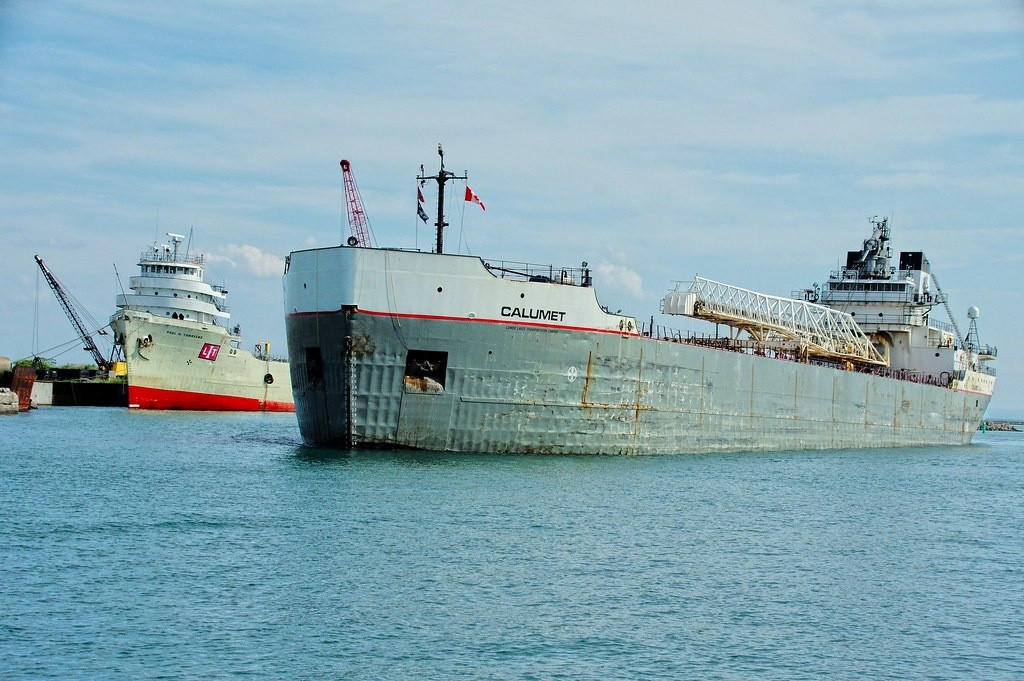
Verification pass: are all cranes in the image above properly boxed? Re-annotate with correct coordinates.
[34,255,116,373]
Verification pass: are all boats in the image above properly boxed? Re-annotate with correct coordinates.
[113,227,295,412]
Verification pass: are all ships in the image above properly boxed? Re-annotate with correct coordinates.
[281,143,999,455]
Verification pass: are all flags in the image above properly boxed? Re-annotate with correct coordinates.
[417,186,429,224]
[464,186,486,211]
